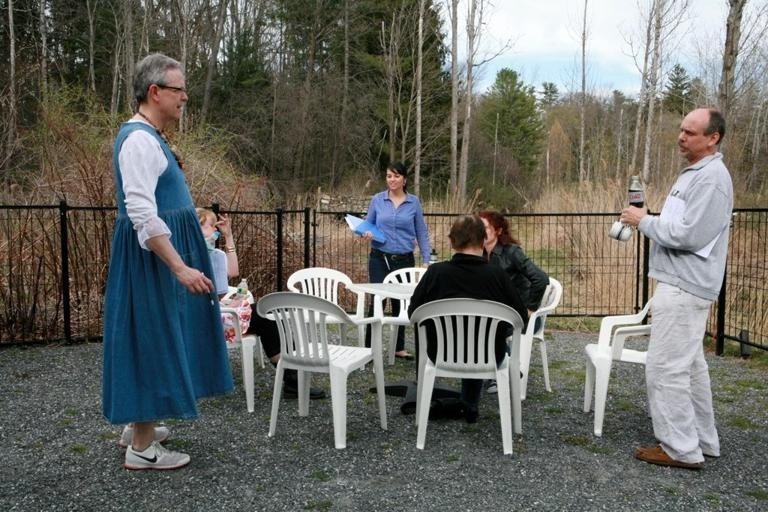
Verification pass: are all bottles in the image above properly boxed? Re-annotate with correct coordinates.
[628,174,645,208]
[237,278,248,298]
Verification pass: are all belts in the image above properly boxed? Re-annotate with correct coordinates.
[370,248,412,262]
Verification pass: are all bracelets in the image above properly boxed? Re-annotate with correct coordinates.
[225,245,235,254]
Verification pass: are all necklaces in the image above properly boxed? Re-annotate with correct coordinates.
[138,111,186,170]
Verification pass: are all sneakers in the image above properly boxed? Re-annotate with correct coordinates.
[633,445,704,470]
[123,442,191,471]
[118,426,171,448]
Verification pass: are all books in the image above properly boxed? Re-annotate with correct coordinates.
[344,213,387,244]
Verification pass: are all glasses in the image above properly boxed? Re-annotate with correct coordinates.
[157,82,189,94]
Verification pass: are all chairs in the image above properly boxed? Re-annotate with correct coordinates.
[519,278,563,402]
[372,268,427,374]
[410,297,524,455]
[287,267,366,372]
[220,288,266,413]
[583,298,650,439]
[256,293,387,451]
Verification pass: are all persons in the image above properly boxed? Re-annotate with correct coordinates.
[407,213,527,423]
[360,161,430,366]
[621,108,735,470]
[193,207,326,400]
[100,55,235,471]
[479,210,550,337]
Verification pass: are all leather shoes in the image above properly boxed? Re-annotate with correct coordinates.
[465,405,479,424]
[397,351,415,360]
[282,384,326,402]
[428,399,467,422]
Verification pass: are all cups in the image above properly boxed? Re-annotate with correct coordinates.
[607,219,633,241]
